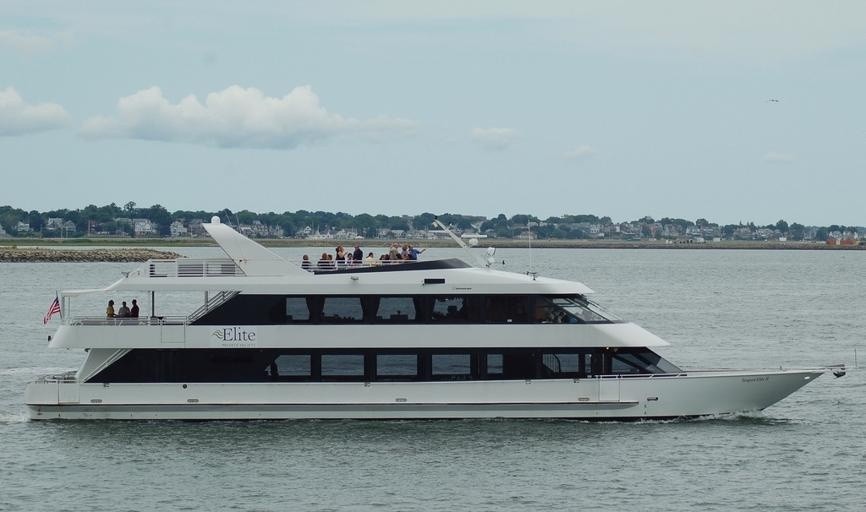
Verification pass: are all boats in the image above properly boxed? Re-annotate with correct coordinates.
[20,214,824,426]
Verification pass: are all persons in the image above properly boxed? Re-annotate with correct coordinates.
[106,300,116,317]
[317,241,426,269]
[118,302,130,317]
[301,255,313,271]
[130,299,139,317]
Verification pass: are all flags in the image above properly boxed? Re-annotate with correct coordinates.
[43,297,61,324]
